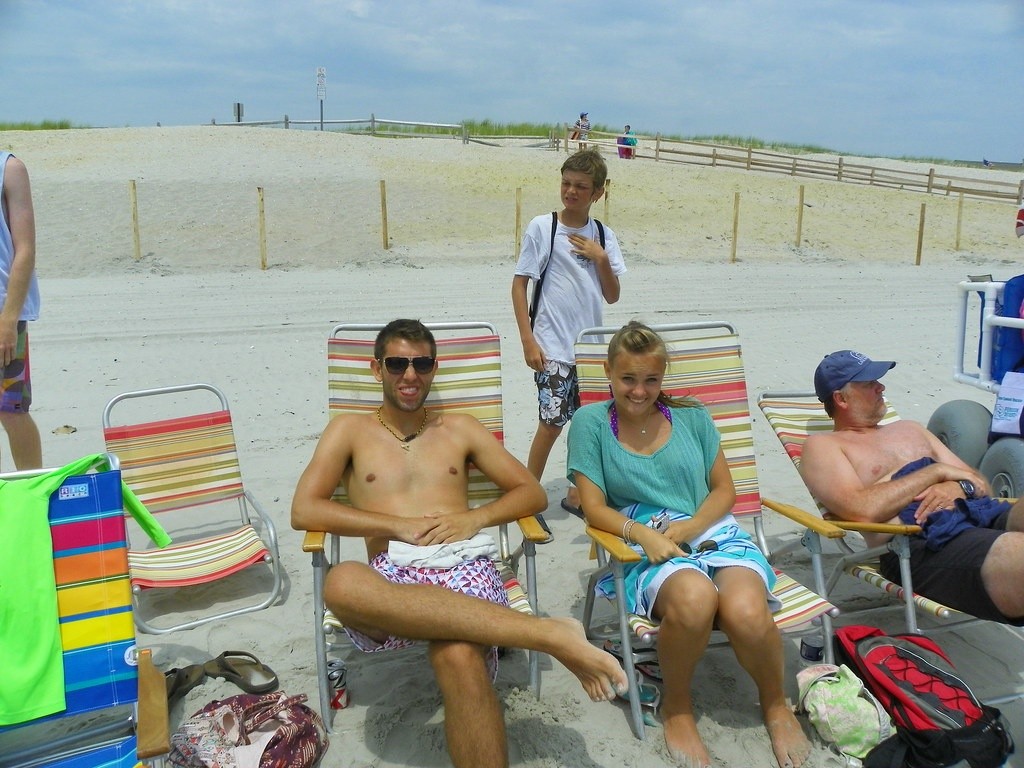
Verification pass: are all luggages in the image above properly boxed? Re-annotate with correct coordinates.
[616,136,632,159]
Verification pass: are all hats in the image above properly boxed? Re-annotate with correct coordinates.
[814,350,896,403]
[580,112,588,117]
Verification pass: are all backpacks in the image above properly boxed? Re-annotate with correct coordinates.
[832,623,1015,768]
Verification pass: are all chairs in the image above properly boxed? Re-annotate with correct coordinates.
[304,322,555,736]
[103,383,280,634]
[0,454,170,768]
[757,390,1024,705]
[575,321,845,741]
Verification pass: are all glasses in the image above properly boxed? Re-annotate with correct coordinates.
[678,540,747,560]
[378,356,437,374]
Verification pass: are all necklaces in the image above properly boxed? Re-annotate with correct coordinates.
[377,404,428,452]
[618,406,651,434]
[627,521,641,546]
[623,519,635,543]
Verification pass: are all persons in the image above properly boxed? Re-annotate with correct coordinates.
[574,112,591,149]
[623,125,636,159]
[801,350,1024,627]
[567,320,812,768]
[512,150,627,545]
[291,318,630,768]
[0,152,43,472]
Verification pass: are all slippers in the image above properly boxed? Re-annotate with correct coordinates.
[164,663,206,711]
[533,514,554,545]
[203,651,279,693]
[606,638,663,680]
[561,497,586,520]
[610,669,662,727]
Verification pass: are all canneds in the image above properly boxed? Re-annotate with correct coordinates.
[326,658,349,709]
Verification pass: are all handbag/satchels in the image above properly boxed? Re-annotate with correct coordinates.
[967,270,1024,385]
[168,687,330,768]
[570,130,580,140]
[986,368,1024,444]
[627,132,638,144]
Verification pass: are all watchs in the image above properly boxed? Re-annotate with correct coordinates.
[957,480,976,500]
